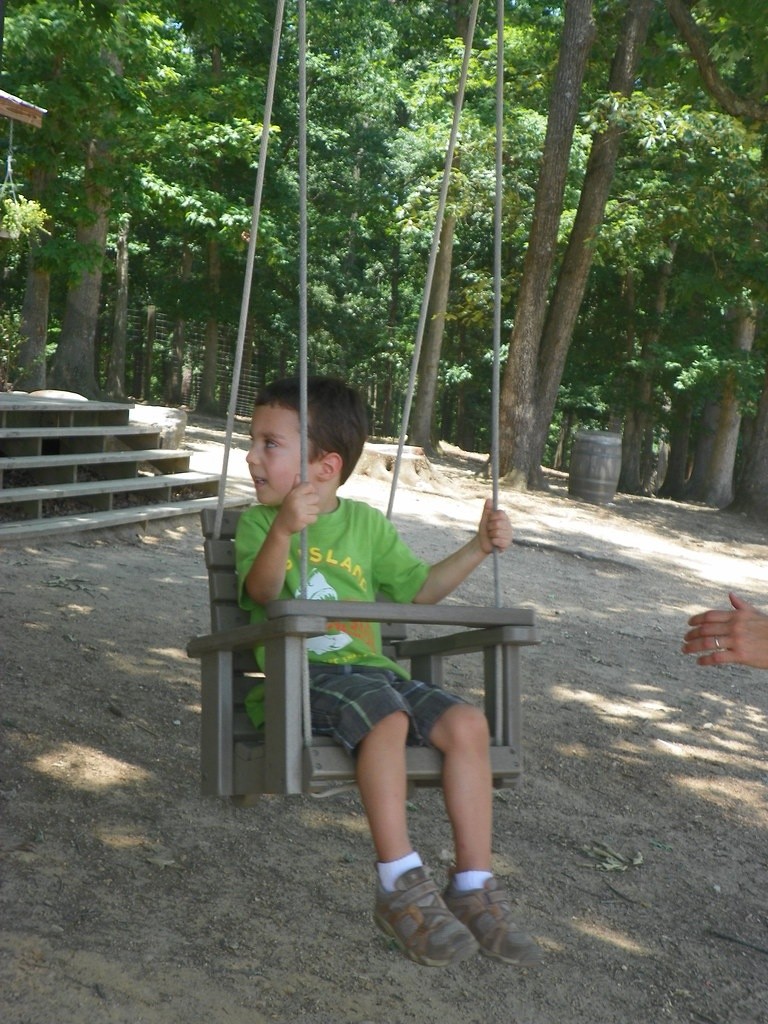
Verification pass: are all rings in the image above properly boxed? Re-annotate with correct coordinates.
[714,636,721,650]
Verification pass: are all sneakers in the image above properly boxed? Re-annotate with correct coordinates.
[372,861,480,968]
[442,866,541,967]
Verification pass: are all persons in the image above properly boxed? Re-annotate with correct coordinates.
[681,591,768,670]
[236,377,543,967]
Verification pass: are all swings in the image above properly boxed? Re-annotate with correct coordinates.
[182,2,541,803]
